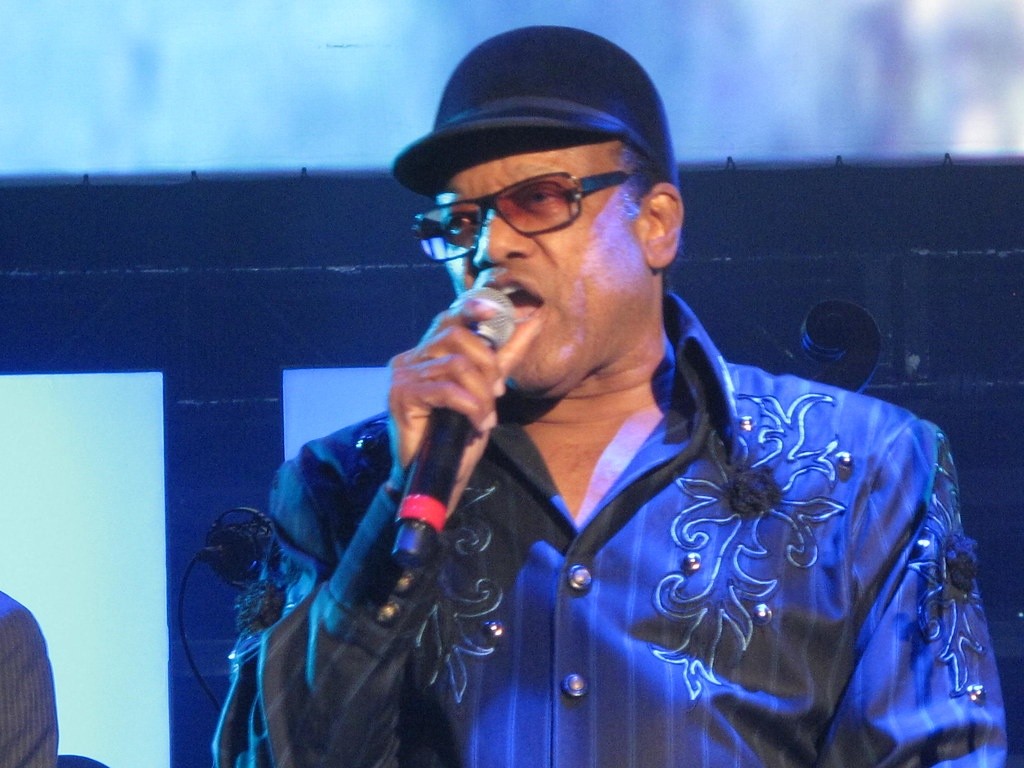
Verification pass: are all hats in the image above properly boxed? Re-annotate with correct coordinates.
[392,24,680,198]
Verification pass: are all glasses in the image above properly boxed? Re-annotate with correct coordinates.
[408,171,667,265]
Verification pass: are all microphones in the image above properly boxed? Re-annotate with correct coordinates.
[391,286,516,569]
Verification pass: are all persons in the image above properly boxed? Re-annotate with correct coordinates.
[212,26,1008,768]
